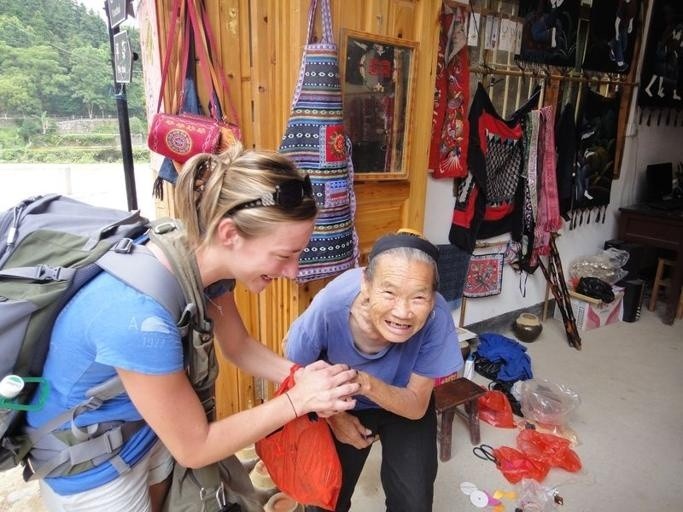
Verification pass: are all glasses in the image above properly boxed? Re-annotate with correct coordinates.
[223,172,313,217]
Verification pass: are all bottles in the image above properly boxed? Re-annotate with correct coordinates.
[234,441,305,512]
[0,374,23,441]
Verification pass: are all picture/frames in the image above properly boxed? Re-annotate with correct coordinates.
[335,27,420,181]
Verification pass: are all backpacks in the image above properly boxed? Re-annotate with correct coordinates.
[0,192,198,482]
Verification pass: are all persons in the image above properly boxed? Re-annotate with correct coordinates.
[277,229,464,512]
[24,147,362,512]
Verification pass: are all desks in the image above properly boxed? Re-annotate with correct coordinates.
[618,203,682,327]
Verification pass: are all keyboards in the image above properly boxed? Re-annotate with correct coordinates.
[648,198,680,210]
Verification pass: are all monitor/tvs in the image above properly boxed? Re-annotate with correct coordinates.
[647,163,672,200]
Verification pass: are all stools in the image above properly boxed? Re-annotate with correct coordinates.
[434,376,488,462]
[648,257,682,321]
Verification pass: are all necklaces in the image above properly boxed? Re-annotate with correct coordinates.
[204,287,225,317]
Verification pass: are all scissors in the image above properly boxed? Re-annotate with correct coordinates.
[472,445,499,466]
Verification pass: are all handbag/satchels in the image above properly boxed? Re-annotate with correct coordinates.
[144,111,242,165]
[165,454,264,512]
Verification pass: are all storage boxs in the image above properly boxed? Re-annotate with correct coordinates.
[553,283,626,331]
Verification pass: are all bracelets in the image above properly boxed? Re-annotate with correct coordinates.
[285,391,298,420]
